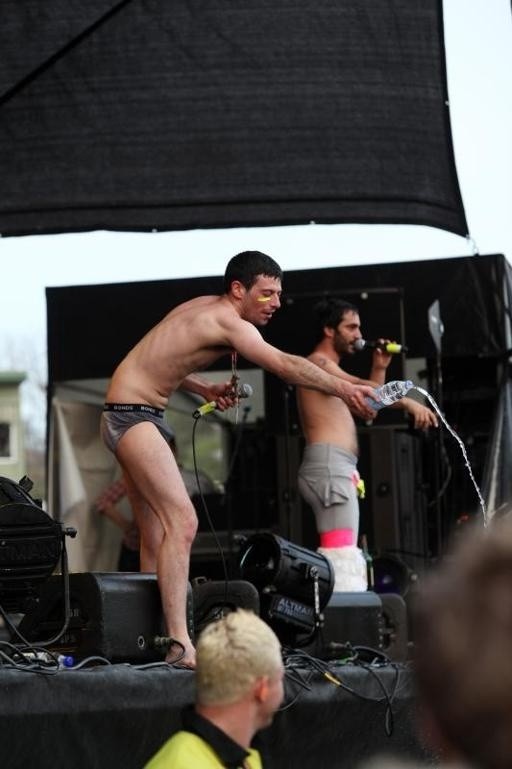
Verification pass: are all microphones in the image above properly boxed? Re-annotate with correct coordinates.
[352,335,412,361]
[190,383,253,419]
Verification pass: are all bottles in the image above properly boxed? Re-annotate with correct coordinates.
[366,380,413,409]
[359,534,374,589]
[13,652,73,668]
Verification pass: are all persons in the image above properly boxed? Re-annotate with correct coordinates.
[294,299,438,549]
[97,470,140,572]
[403,515,512,769]
[141,610,286,769]
[99,251,380,672]
[167,437,217,496]
